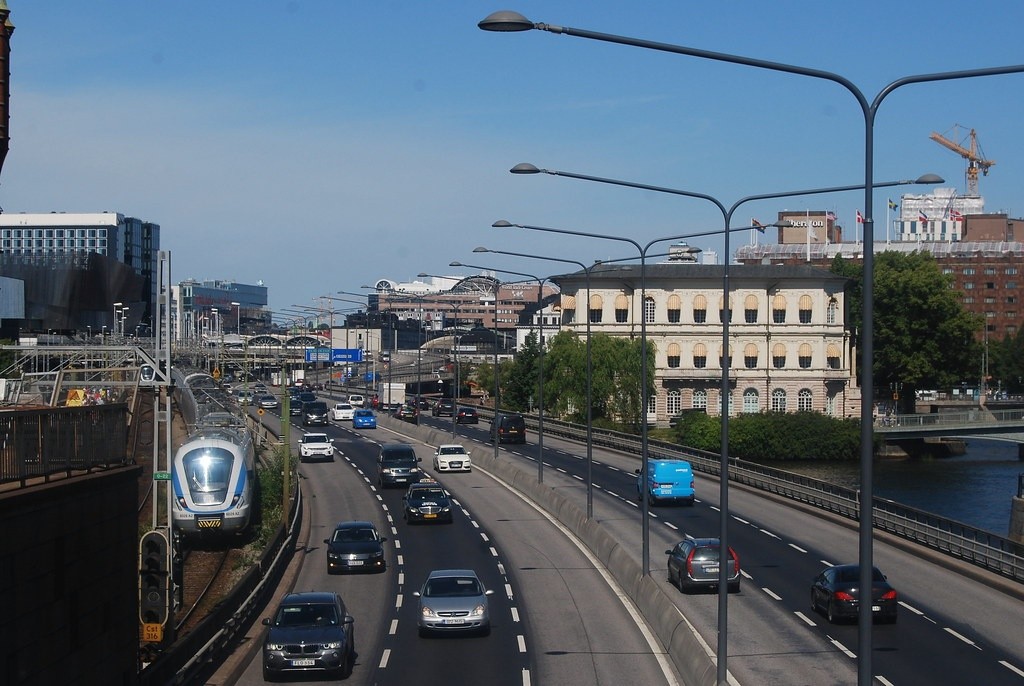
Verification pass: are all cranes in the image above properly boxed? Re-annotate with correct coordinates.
[929,115,994,200]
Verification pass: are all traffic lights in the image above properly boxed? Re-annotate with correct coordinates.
[138,530,170,628]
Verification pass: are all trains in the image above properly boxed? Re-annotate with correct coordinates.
[163,360,258,546]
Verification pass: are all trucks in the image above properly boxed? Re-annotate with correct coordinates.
[376,382,407,413]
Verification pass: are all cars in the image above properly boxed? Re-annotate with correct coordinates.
[413,569,495,639]
[258,394,278,410]
[261,590,356,682]
[237,390,254,406]
[297,432,334,463]
[664,536,741,595]
[433,443,472,473]
[810,561,899,626]
[329,404,357,421]
[392,404,420,424]
[348,395,364,408]
[401,477,455,524]
[351,409,378,429]
[322,520,386,576]
[407,397,429,411]
[453,407,478,424]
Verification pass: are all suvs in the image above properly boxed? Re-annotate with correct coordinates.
[431,398,455,417]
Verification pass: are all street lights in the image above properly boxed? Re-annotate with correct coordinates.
[448,261,633,486]
[478,7,1024,686]
[507,160,946,686]
[202,284,525,440]
[417,272,569,460]
[493,215,793,577]
[472,245,702,522]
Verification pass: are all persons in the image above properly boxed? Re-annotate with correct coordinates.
[479,397,484,405]
[486,394,489,400]
[988,387,999,401]
[873,402,879,422]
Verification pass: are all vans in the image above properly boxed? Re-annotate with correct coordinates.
[488,413,526,444]
[634,458,695,507]
[376,443,423,489]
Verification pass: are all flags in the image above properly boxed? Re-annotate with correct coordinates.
[889,198,898,212]
[827,211,839,221]
[950,208,964,221]
[856,211,865,223]
[810,224,818,241]
[919,210,927,222]
[752,218,766,234]
